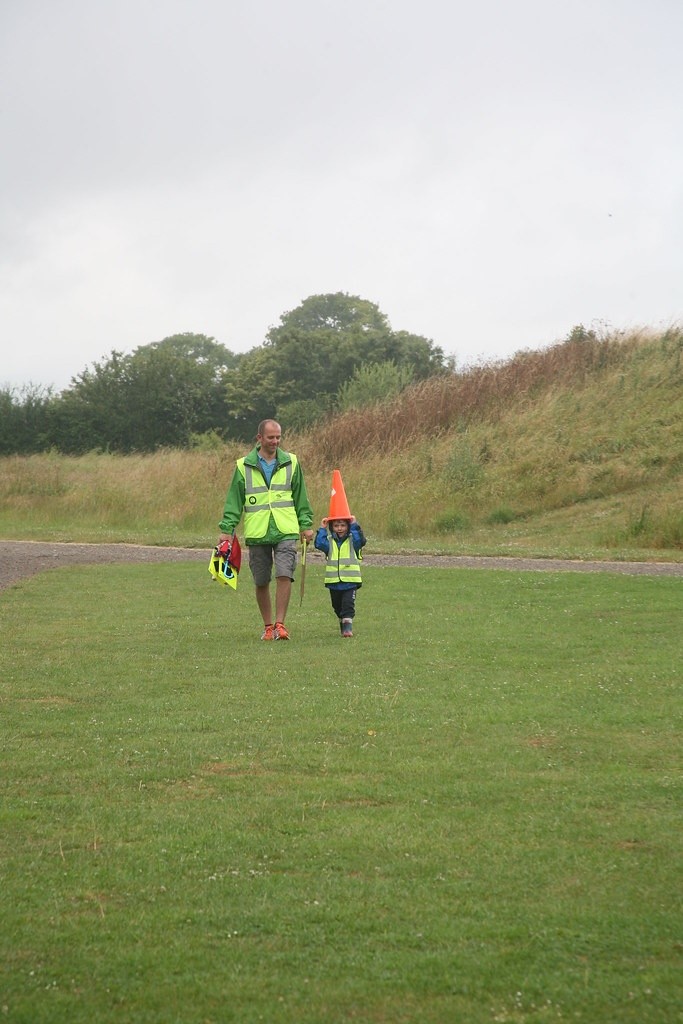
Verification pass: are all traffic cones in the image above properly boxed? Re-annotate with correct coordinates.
[323,469,352,521]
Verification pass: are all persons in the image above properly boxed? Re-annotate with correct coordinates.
[314,515,368,639]
[216,418,314,642]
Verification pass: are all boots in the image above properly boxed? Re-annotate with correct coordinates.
[338,617,353,637]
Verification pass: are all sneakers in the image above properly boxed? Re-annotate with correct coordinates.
[261,624,274,641]
[274,622,290,640]
